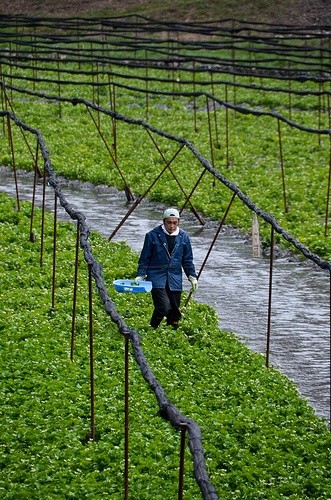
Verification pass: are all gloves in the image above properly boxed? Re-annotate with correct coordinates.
[189,276,198,292]
[135,276,146,284]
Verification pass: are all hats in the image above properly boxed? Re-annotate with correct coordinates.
[164,208,179,219]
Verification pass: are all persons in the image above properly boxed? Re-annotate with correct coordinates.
[135,208,199,330]
